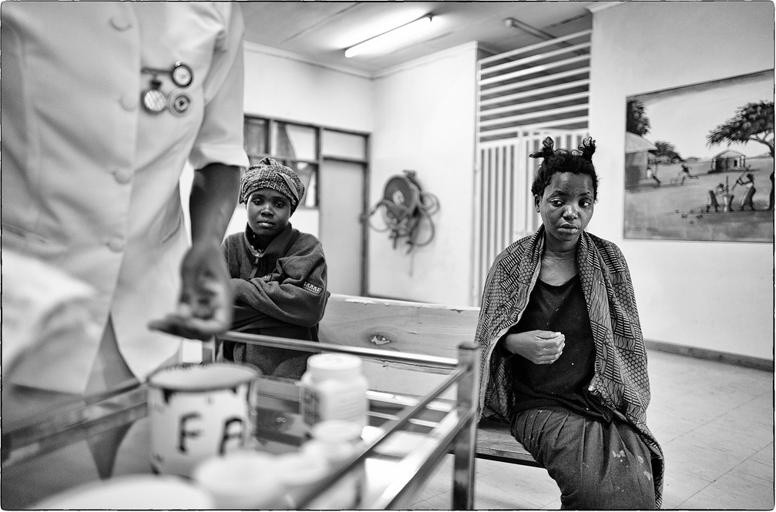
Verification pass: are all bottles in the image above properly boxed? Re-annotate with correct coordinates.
[296,351,369,443]
[191,417,368,509]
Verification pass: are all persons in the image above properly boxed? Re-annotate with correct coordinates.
[215,155,329,388]
[0,0,248,509]
[474,142,667,510]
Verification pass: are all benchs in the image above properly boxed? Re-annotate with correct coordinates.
[237,294,549,468]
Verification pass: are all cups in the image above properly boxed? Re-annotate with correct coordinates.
[144,359,262,480]
